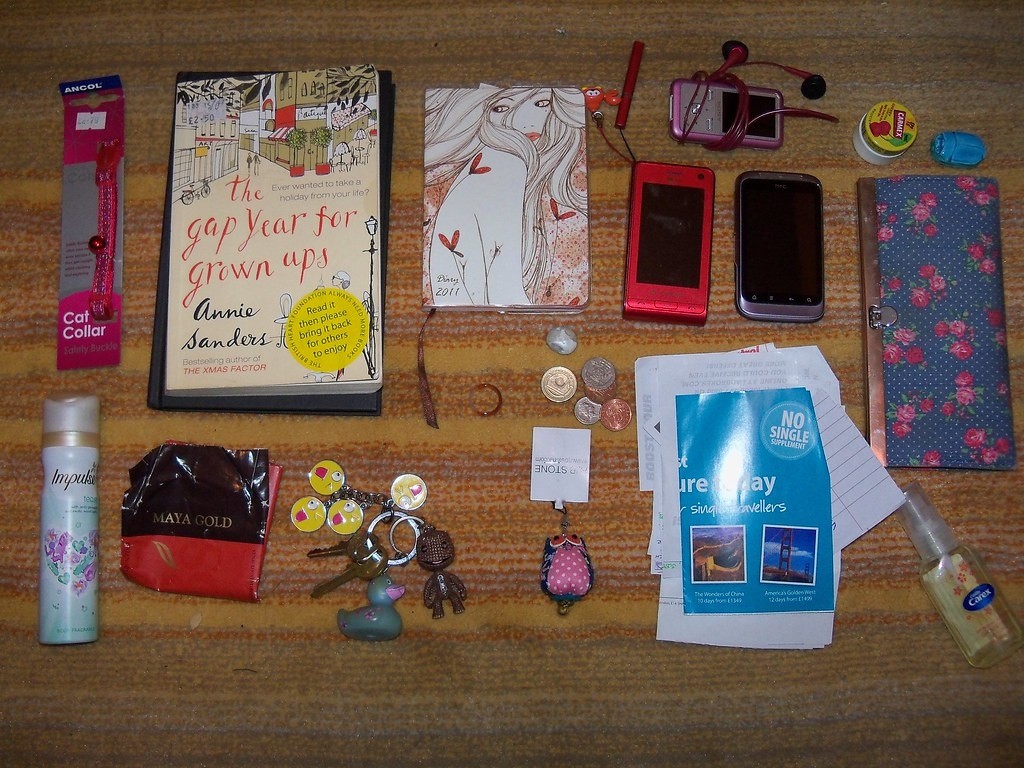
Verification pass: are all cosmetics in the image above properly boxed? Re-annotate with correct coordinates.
[895,482,1024,669]
[37,394,101,643]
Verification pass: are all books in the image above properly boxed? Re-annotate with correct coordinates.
[422,85,588,311]
[148,64,395,416]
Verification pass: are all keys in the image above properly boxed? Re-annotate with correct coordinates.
[307,532,379,563]
[311,546,388,599]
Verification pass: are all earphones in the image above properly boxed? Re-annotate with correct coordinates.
[720,40,749,75]
[784,65,826,99]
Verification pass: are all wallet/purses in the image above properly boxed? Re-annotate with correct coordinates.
[856,172,1017,472]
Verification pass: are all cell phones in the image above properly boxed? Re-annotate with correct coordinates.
[734,169,824,323]
[622,160,716,328]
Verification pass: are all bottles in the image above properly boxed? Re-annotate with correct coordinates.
[894,480,1024,667]
